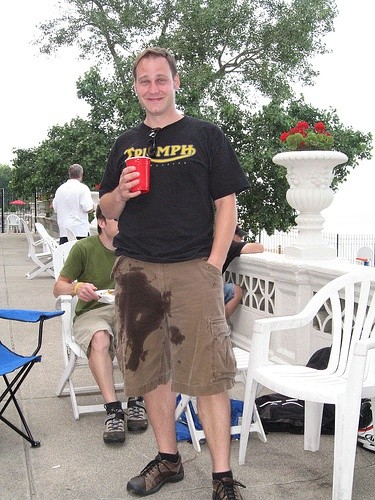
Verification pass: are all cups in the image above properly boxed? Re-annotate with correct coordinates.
[125,156,151,194]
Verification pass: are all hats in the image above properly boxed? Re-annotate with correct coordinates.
[235,225,247,236]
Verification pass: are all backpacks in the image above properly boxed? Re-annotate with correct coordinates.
[258,347,373,435]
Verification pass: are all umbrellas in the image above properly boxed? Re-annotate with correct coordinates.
[10,200,27,212]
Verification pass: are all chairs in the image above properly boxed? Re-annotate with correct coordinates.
[240,265,375,500]
[54,240,125,421]
[20,218,60,279]
[7,214,21,234]
[0,310,65,449]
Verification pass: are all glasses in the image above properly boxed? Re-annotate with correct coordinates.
[147,126,161,156]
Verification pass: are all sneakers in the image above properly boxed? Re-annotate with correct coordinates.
[127,394,147,430]
[103,406,126,443]
[126,454,185,496]
[211,478,246,500]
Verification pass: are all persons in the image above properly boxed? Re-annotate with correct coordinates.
[52,205,147,444]
[51,164,95,244]
[99,47,249,500]
[222,226,265,328]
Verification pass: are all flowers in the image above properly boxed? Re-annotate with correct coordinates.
[279,122,334,149]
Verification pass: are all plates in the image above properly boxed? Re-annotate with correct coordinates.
[94,289,115,299]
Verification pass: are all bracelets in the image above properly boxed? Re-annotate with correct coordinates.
[73,282,83,295]
